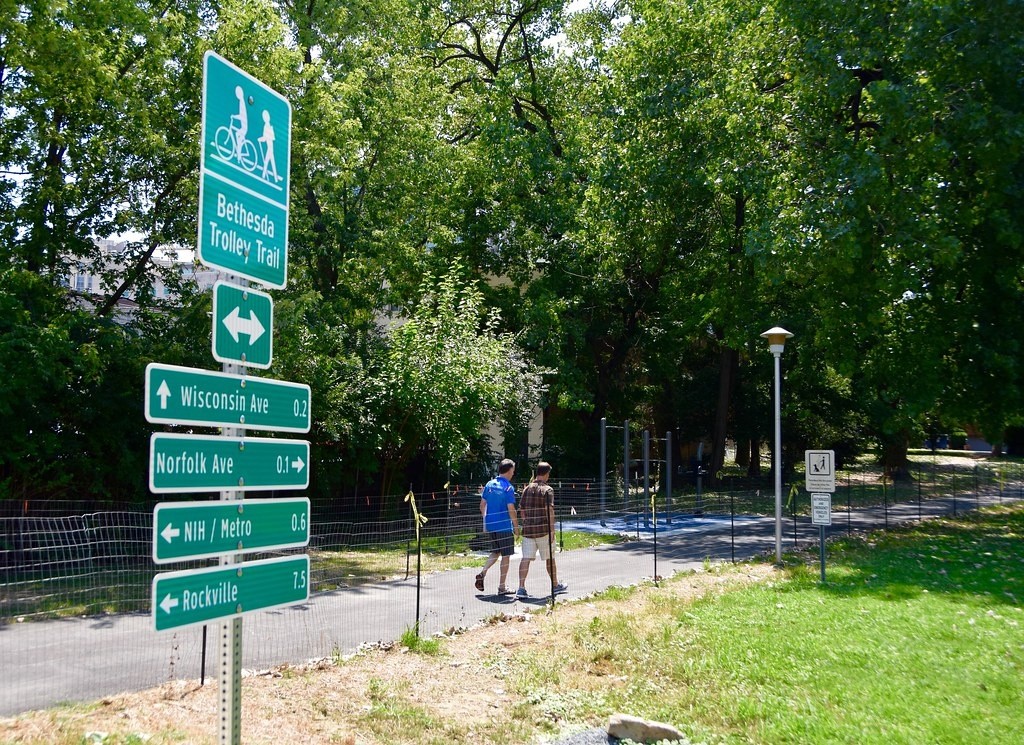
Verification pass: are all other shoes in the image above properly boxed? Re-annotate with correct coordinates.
[498,585,516,595]
[475,573,485,591]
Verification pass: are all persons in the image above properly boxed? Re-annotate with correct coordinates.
[475,459,520,595]
[514,463,568,599]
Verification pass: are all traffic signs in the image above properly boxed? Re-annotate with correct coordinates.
[144,365,312,635]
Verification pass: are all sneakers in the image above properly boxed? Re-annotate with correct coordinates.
[553,583,568,594]
[516,588,533,598]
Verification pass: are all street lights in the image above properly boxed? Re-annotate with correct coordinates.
[759,326,795,563]
